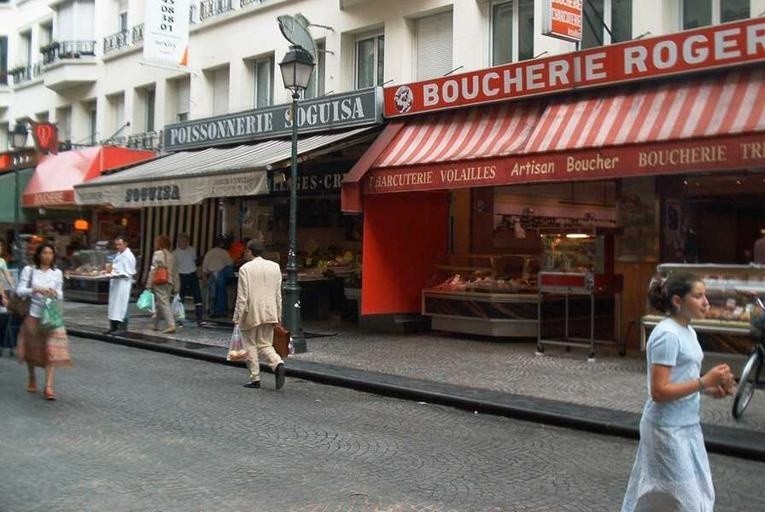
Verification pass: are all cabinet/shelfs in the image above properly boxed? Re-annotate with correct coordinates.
[641,261,765,384]
[62,247,144,305]
[420,253,540,342]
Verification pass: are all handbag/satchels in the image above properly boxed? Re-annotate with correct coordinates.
[40,295,63,329]
[7,295,32,317]
[154,267,168,285]
[273,326,290,357]
[137,289,152,310]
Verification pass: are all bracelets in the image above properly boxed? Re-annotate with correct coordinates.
[696,376,705,393]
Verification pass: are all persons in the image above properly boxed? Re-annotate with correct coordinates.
[14,243,72,400]
[623,272,739,511]
[0,230,248,357]
[231,237,286,389]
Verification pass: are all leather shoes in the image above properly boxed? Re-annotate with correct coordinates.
[243,383,260,388]
[276,363,286,389]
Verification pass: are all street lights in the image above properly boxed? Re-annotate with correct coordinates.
[277,45,317,355]
[5,123,29,269]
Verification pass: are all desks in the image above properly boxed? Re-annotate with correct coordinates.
[297,276,338,320]
[537,271,624,360]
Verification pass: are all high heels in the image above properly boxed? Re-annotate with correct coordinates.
[28,383,37,392]
[44,385,56,399]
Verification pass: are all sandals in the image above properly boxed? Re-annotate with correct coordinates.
[164,327,176,333]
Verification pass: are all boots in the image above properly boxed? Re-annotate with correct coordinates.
[110,319,126,335]
[195,305,207,327]
[103,319,120,334]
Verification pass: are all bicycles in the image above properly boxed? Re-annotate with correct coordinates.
[730,284,764,422]
